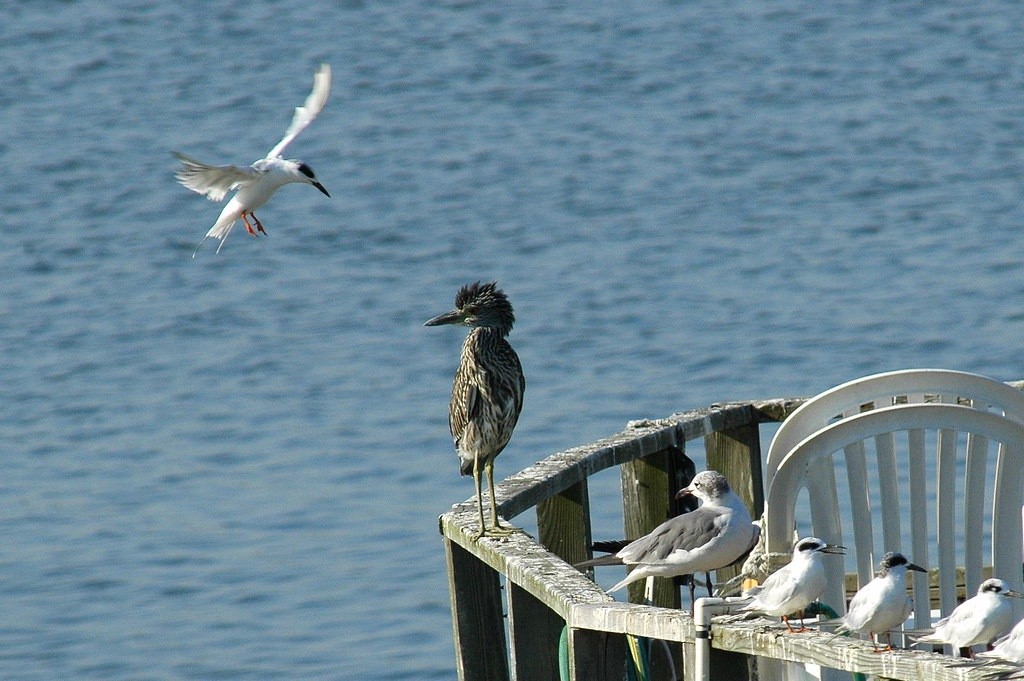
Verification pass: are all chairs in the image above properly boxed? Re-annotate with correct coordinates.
[761,369,1024,680]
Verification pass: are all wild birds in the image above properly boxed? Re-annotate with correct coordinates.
[906,578,1024,681]
[172,63,331,259]
[824,551,928,652]
[423,280,535,543]
[573,470,849,634]
